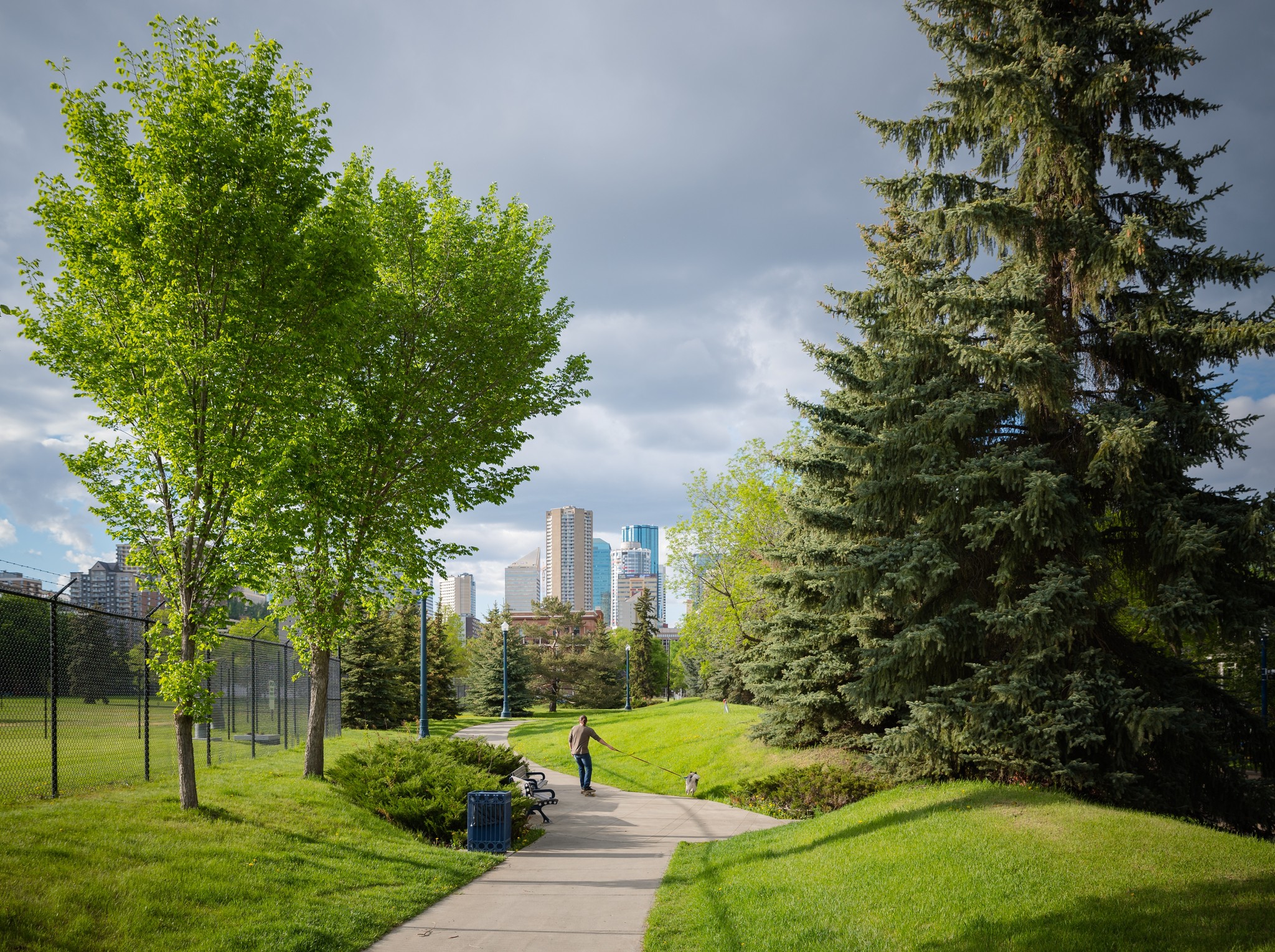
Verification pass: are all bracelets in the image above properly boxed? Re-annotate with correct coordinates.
[571,750,572,751]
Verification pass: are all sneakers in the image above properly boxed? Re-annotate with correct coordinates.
[581,787,595,792]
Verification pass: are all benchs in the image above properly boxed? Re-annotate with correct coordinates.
[508,764,559,824]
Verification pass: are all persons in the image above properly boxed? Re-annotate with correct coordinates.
[568,715,613,792]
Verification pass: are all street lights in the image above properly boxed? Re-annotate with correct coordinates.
[624,644,632,710]
[501,621,512,717]
[415,574,432,741]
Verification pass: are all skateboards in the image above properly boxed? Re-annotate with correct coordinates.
[579,786,596,796]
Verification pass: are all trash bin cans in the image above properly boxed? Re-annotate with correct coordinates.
[468,790,511,853]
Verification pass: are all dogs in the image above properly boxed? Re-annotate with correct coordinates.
[684,770,700,796]
